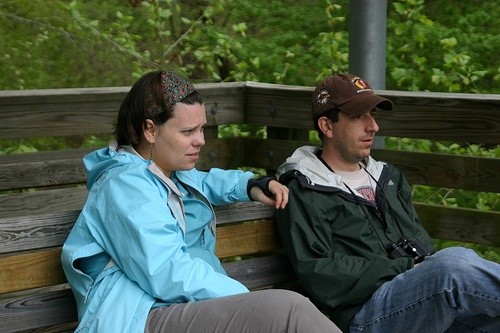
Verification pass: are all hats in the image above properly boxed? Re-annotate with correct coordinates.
[311,74,394,121]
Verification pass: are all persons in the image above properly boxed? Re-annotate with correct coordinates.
[272,73,500,333]
[61,71,343,333]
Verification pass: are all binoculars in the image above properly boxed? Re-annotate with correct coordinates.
[385,235,427,264]
